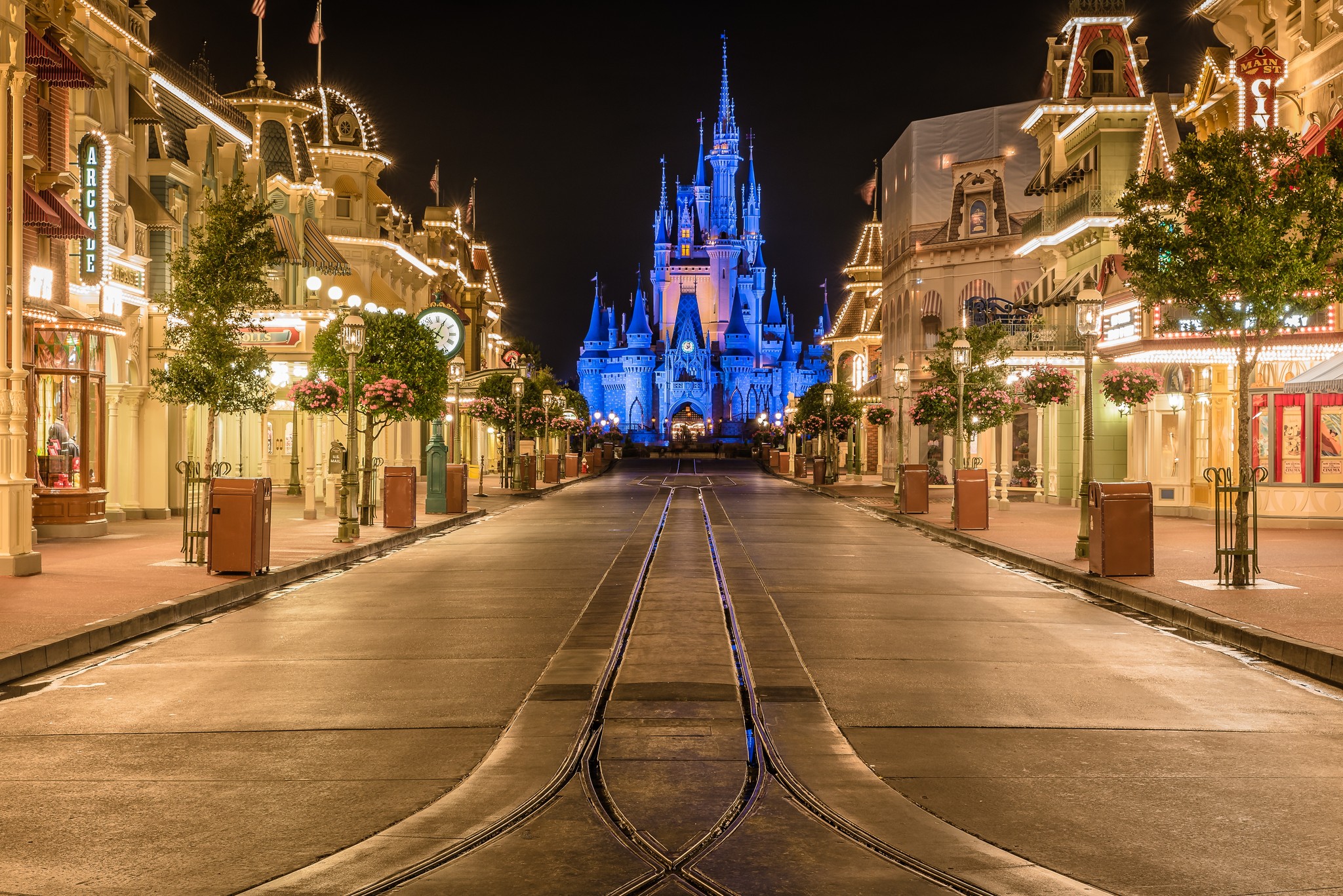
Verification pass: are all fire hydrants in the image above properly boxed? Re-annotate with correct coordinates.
[581,455,590,475]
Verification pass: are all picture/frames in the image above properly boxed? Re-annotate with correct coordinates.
[47,438,61,456]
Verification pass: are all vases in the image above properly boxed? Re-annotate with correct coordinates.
[929,476,934,484]
[1031,484,1036,487]
[1010,484,1021,487]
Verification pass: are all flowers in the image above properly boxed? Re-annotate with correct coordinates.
[928,464,949,485]
[287,354,625,440]
[1010,464,1043,485]
[752,362,1165,442]
[1027,312,1046,326]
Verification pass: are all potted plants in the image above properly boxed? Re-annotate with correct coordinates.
[1018,429,1031,487]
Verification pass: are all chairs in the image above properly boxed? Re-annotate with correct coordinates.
[997,329,1057,351]
[1170,432,1179,476]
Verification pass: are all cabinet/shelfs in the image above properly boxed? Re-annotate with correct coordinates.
[37,456,73,487]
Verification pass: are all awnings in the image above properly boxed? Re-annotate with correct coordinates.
[7,173,95,240]
[921,279,997,319]
[1260,109,1343,197]
[129,83,166,126]
[268,215,352,276]
[128,174,181,231]
[1023,145,1098,196]
[334,269,406,308]
[1003,379,1038,405]
[34,303,125,336]
[1283,351,1343,393]
[24,21,108,90]
[498,358,508,368]
[1012,265,1097,308]
[430,288,471,326]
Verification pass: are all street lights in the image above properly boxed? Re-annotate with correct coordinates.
[1073,272,1107,561]
[445,352,465,465]
[824,384,834,484]
[541,381,555,481]
[506,366,529,489]
[892,355,915,509]
[588,405,620,447]
[665,417,669,431]
[338,304,366,538]
[707,417,711,431]
[757,404,786,448]
[651,416,656,430]
[557,390,567,479]
[948,329,973,524]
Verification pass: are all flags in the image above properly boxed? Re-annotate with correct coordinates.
[251,0,265,18]
[308,9,324,44]
[466,187,473,224]
[430,165,437,193]
[860,170,876,205]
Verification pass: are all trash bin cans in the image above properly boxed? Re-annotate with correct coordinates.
[545,454,561,483]
[382,466,416,528]
[592,448,602,466]
[761,443,770,461]
[614,445,623,459]
[584,452,594,474]
[813,455,827,484]
[794,454,807,478]
[1087,482,1155,578]
[603,442,613,460]
[751,448,760,459]
[519,455,536,489]
[565,453,578,477]
[954,468,989,531]
[899,463,929,515]
[208,476,272,577]
[446,463,468,515]
[770,448,779,466]
[779,452,790,473]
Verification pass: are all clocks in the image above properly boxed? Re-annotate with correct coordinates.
[415,307,466,361]
[681,340,695,353]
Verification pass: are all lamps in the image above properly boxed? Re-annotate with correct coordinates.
[1118,401,1131,418]
[444,414,453,426]
[306,276,407,317]
[651,416,723,428]
[1165,379,1180,414]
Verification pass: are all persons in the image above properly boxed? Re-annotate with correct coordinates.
[48,415,79,458]
[680,422,688,444]
[496,434,504,469]
[714,439,723,459]
